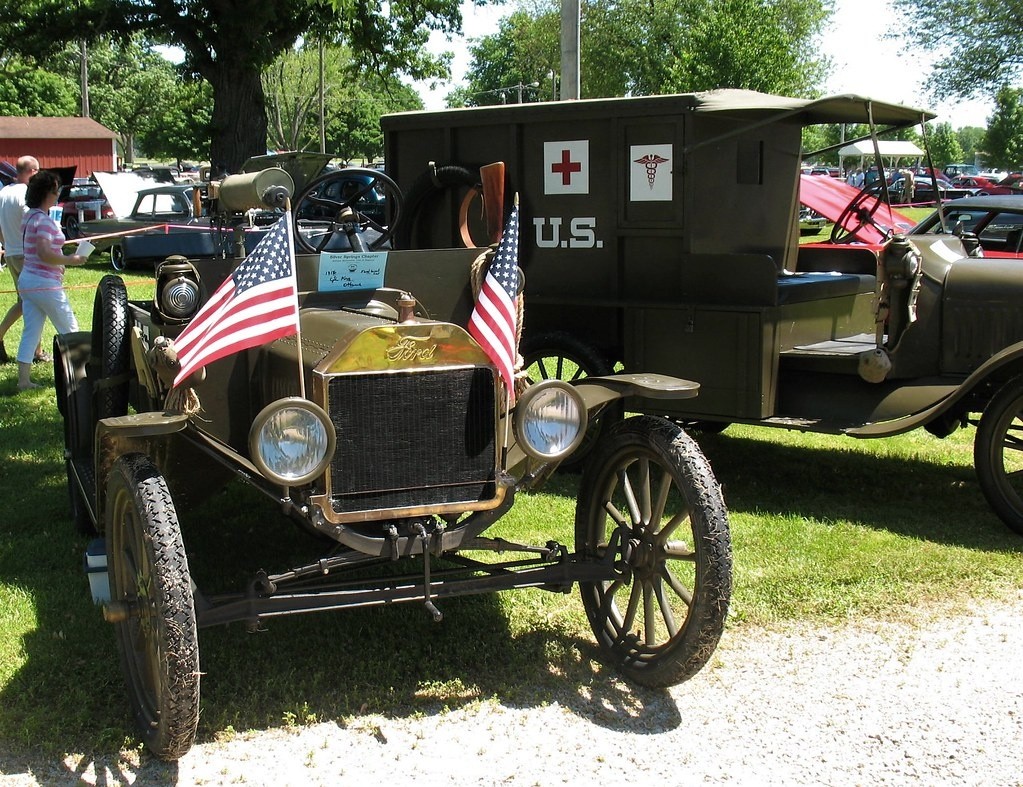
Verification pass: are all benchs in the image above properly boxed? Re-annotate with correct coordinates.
[682,246,879,306]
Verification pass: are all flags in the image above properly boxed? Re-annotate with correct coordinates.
[467,205,520,403]
[173,210,299,388]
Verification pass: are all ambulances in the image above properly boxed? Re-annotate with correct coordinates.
[378,82,1022,534]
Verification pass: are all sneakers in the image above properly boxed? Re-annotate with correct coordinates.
[0,341,9,362]
[33,350,53,363]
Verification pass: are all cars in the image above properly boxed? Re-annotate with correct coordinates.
[122,146,385,191]
[75,183,213,253]
[48,170,736,758]
[901,192,1022,262]
[795,159,1023,201]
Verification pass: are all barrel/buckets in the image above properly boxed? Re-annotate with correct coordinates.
[82,550,112,607]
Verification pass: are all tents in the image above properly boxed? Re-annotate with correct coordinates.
[836,139,924,169]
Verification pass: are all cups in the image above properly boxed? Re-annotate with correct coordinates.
[75,241,96,265]
[50,206,63,227]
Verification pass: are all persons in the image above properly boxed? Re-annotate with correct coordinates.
[16,171,80,389]
[0,155,53,365]
[892,167,914,209]
[843,167,876,187]
